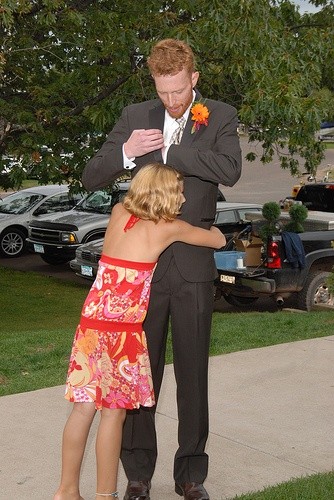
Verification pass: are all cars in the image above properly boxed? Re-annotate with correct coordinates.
[25,179,226,264]
[0,183,84,258]
[69,201,263,283]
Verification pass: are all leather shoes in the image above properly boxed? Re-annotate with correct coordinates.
[123,479,151,500]
[175,481,210,500]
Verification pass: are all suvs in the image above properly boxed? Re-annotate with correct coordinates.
[213,181,334,312]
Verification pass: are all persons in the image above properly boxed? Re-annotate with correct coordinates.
[54,163,226,500]
[81,41,242,500]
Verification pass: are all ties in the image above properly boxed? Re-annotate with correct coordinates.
[169,116,187,145]
